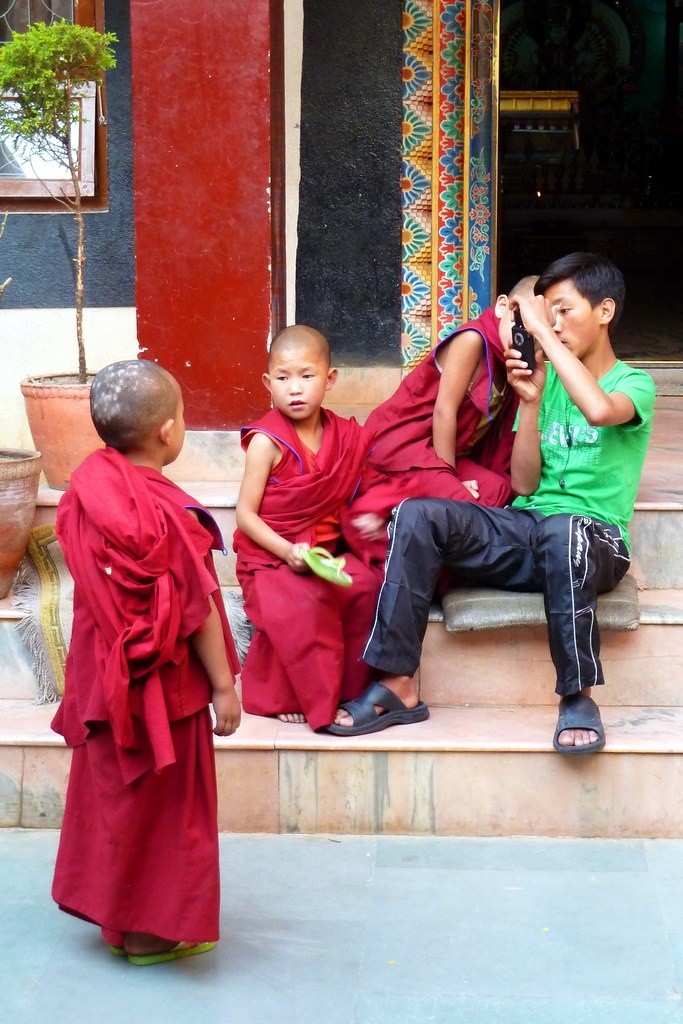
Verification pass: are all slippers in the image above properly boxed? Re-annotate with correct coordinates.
[110,940,219,964]
[554,694,607,756]
[324,680,430,734]
[300,546,353,588]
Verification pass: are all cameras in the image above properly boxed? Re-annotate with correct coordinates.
[509,308,536,370]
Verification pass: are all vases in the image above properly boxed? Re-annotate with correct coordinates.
[0,448,42,600]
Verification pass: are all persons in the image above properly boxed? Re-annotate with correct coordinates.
[309,251,656,759]
[230,326,401,731]
[50,361,242,968]
[362,273,548,506]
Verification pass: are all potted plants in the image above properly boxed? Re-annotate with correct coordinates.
[0,18,119,492]
